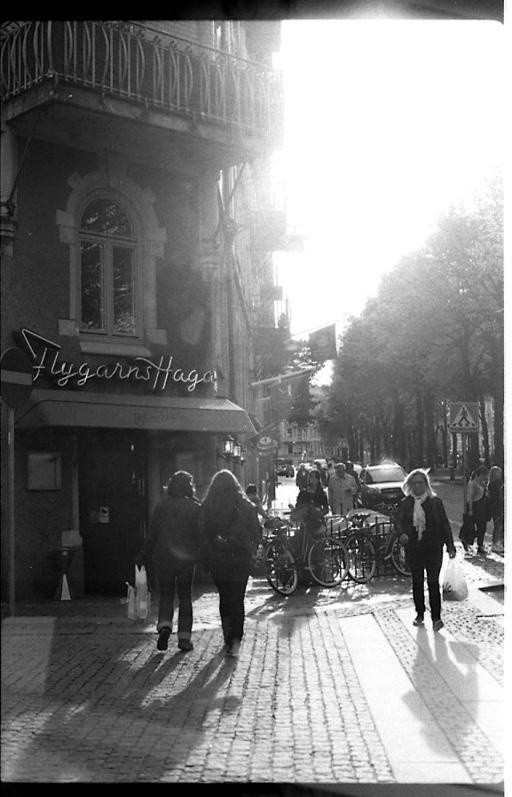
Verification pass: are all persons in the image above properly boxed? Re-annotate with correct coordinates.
[196,468,261,657]
[392,466,457,631]
[136,470,200,651]
[245,480,267,523]
[457,465,491,557]
[486,465,503,552]
[284,455,361,519]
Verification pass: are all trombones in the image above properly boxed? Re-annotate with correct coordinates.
[447,400,481,434]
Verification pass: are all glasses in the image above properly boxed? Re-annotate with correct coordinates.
[217,434,246,467]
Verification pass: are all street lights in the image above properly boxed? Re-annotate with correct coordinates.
[478,504,491,522]
[459,517,475,545]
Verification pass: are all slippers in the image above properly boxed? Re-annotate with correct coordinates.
[202,501,251,569]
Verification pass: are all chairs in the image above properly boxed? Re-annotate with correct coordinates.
[222,639,242,659]
[156,627,171,651]
[177,638,194,652]
[492,543,504,552]
[432,619,444,633]
[465,543,477,555]
[477,545,490,555]
[413,613,424,626]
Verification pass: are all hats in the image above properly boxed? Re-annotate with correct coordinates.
[275,454,413,516]
